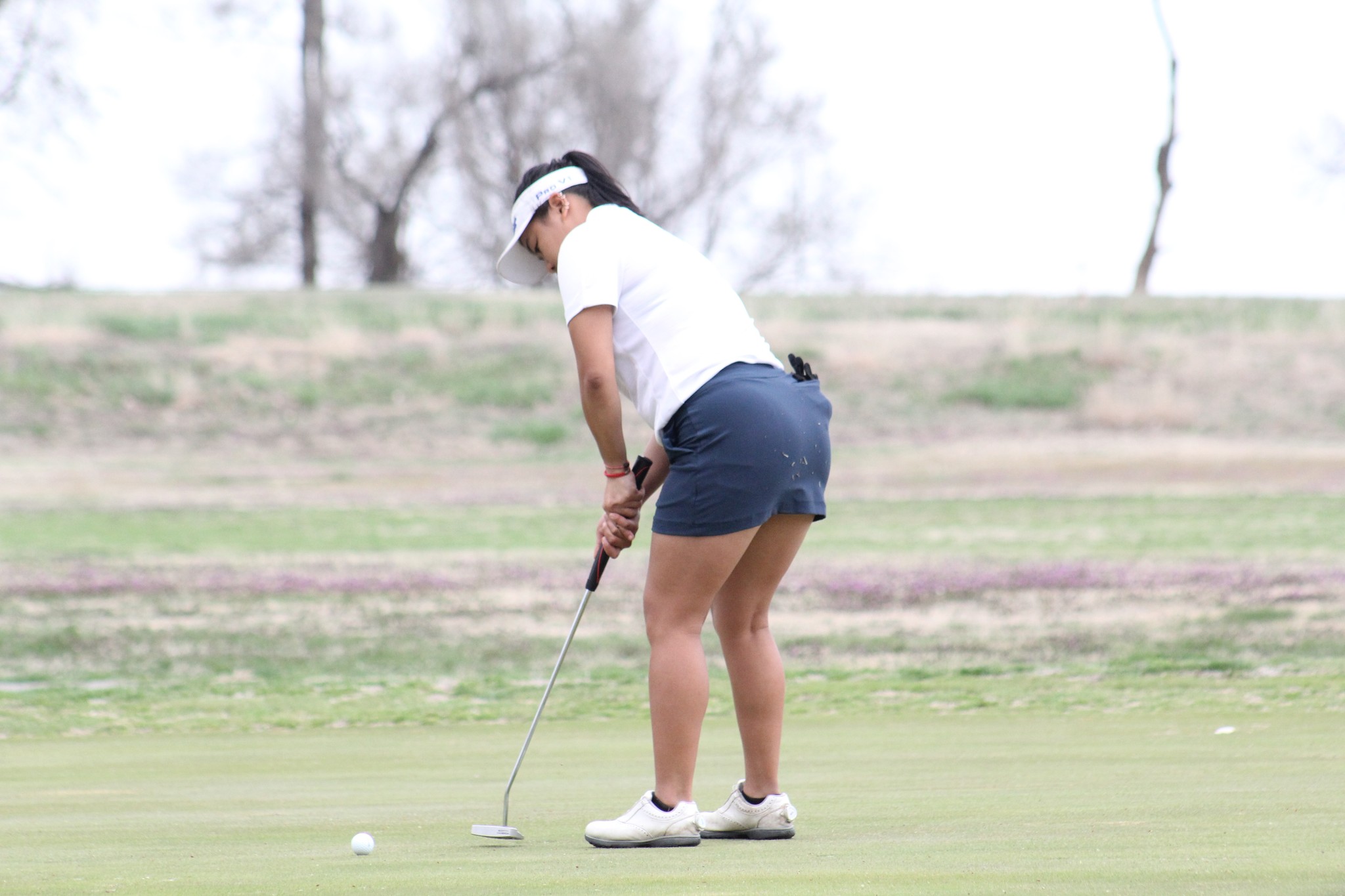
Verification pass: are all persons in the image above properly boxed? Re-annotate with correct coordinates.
[494,151,833,847]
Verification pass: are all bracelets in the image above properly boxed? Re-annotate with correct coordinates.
[604,462,631,478]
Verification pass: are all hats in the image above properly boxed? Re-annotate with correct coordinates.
[496,165,588,286]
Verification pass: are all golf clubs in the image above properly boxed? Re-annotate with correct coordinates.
[469,448,655,844]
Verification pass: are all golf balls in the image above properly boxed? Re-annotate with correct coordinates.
[350,832,375,856]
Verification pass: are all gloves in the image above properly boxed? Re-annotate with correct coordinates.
[788,354,818,382]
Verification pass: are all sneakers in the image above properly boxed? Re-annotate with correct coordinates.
[700,779,797,839]
[584,790,701,848]
